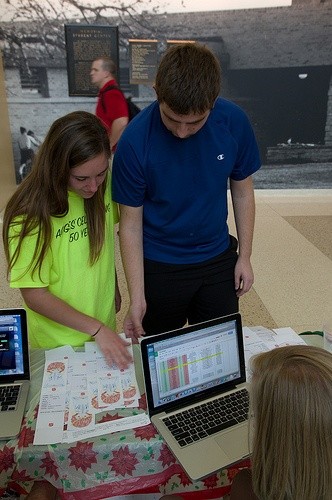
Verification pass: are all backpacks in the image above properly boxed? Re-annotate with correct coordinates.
[101,85,142,126]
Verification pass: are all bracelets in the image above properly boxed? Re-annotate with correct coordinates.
[91,323,104,338]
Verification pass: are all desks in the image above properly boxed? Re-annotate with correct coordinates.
[0,335,332,500]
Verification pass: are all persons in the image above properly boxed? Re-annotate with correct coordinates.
[111,43,262,343]
[2,111,134,371]
[231,345,332,500]
[17,126,42,171]
[90,55,130,161]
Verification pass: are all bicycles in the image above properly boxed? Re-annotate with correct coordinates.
[18,157,32,181]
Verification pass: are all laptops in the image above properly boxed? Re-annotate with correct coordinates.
[140,313,255,483]
[0,309,30,441]
